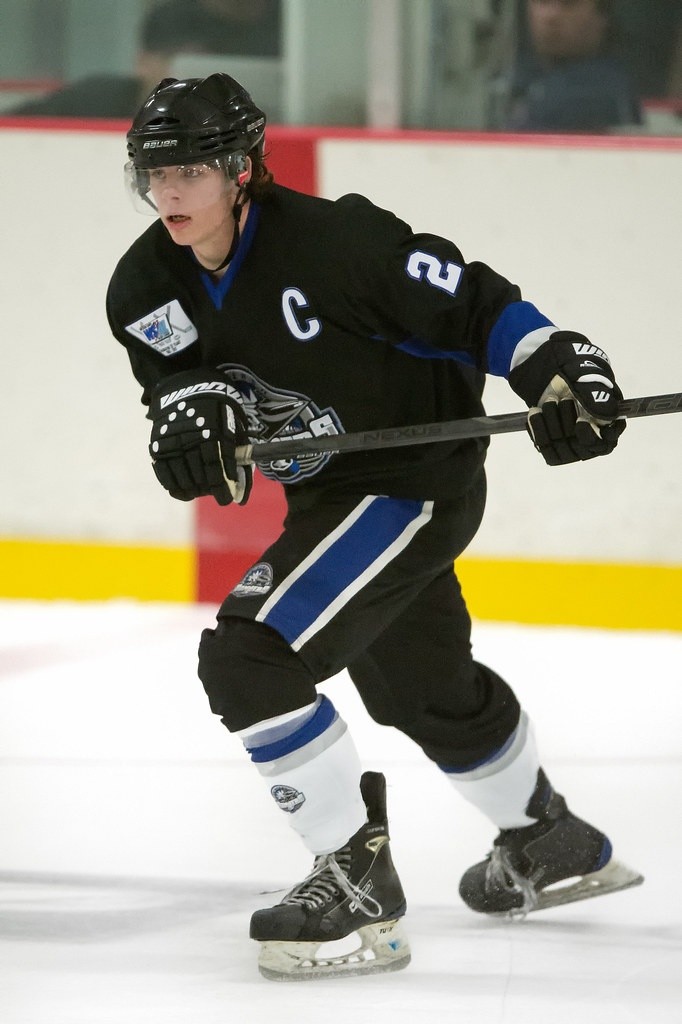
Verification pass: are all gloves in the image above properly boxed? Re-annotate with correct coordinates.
[143,365,257,507]
[506,327,629,466]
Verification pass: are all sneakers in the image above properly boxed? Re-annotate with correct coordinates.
[248,771,413,982]
[458,771,649,920]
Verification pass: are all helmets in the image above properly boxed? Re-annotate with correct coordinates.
[123,68,270,217]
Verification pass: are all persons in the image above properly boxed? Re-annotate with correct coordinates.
[105,73,645,979]
